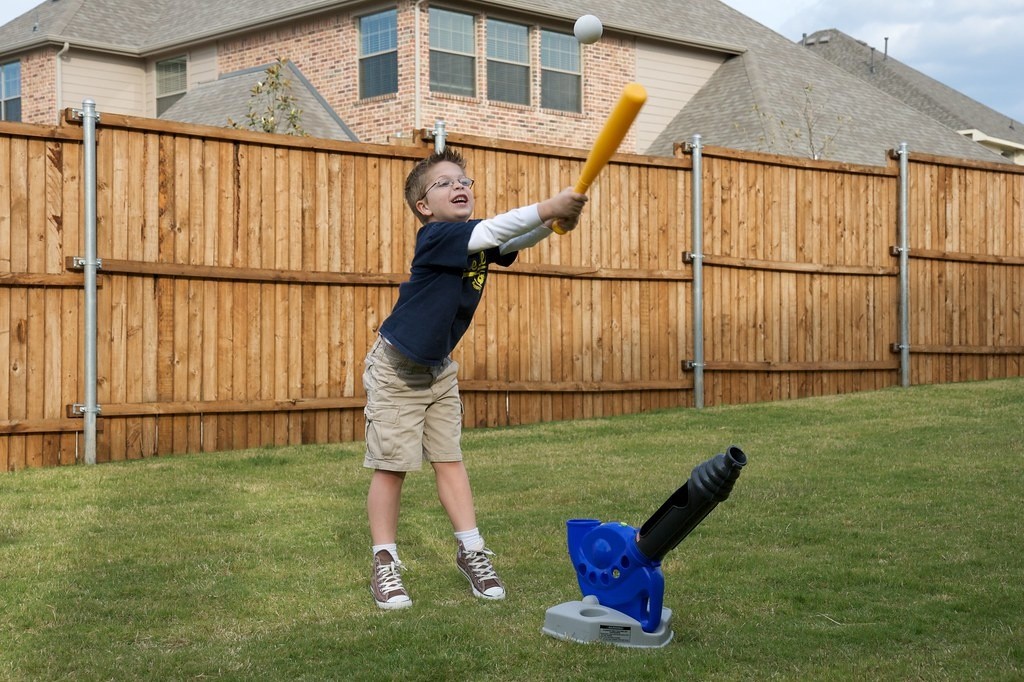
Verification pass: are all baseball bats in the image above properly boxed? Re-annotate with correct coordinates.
[551,84,649,235]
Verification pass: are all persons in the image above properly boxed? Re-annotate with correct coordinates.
[363,146,588,609]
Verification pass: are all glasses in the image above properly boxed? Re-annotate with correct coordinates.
[420,178,474,200]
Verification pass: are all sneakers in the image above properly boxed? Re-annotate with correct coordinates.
[370,549,411,611]
[456,539,505,601]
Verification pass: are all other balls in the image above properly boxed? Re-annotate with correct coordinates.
[572,14,604,45]
